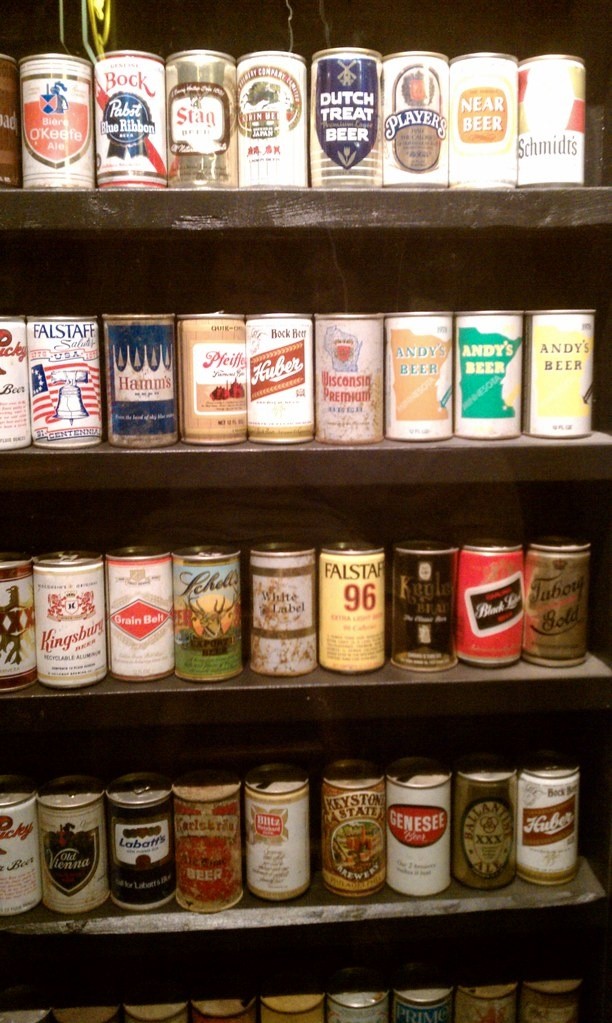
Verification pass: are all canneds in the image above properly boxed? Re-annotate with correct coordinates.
[0,46,588,194]
[0,307,596,454]
[0,534,593,694]
[0,749,584,1023]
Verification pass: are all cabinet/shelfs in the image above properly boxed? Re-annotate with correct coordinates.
[1,0,612,1012]
